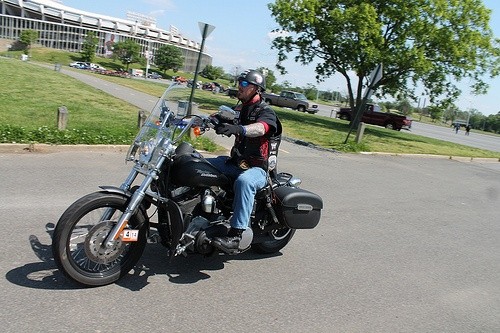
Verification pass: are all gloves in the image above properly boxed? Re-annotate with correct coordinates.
[214,123,243,138]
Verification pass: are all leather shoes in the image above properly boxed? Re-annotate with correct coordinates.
[211,234,242,254]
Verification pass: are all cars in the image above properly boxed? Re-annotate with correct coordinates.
[142,72,162,80]
[202,81,215,90]
[451,119,471,128]
[89,63,126,78]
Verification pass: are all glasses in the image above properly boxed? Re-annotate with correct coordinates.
[239,80,249,87]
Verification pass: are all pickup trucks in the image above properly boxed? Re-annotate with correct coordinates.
[262,88,320,115]
[171,75,188,84]
[227,88,239,98]
[337,102,413,132]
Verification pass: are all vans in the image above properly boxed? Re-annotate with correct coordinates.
[71,61,90,69]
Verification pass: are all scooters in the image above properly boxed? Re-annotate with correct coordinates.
[186,78,200,89]
[211,82,230,95]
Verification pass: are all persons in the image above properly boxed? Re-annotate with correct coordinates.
[197,70,283,255]
[454,122,460,133]
[465,125,470,135]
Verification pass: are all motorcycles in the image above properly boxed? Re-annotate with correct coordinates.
[51,76,325,287]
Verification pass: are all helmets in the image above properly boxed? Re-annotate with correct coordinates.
[237,70,266,91]
